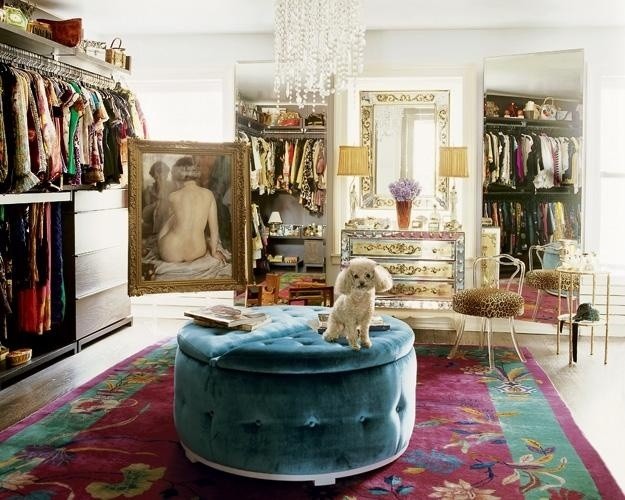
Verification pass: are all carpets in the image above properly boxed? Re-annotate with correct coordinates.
[235,272,325,306]
[499,283,578,324]
[1,338,625,500]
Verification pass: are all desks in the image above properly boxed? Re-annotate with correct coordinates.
[556,267,611,368]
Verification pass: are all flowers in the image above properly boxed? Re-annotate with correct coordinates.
[388,177,421,200]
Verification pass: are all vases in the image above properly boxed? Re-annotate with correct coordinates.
[396,201,412,228]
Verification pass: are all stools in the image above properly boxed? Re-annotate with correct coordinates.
[543,242,581,297]
[174,305,418,487]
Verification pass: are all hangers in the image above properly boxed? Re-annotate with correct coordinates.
[0,43,115,89]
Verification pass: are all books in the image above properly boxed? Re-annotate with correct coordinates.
[184,304,270,331]
[308,314,392,334]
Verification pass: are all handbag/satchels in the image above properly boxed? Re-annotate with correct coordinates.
[304,112,325,126]
[83,37,107,62]
[277,111,301,128]
[105,37,127,68]
[538,96,557,121]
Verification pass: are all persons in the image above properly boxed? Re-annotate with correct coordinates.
[144,162,170,223]
[155,157,225,264]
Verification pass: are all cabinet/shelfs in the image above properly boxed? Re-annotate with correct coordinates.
[237,114,326,274]
[341,230,465,331]
[483,91,583,279]
[0,22,132,383]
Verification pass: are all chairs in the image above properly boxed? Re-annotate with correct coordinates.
[447,254,525,370]
[526,244,579,321]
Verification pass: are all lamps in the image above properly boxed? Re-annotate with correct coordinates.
[337,146,368,218]
[439,147,469,220]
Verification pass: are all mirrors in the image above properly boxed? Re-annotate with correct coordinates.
[477,49,589,335]
[360,90,450,210]
[234,60,335,305]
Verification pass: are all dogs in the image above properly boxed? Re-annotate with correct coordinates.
[322,257,393,352]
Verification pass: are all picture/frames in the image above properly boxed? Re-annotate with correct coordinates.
[129,139,246,297]
